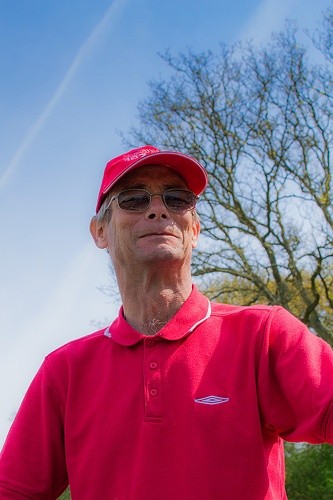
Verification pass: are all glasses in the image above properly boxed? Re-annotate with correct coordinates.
[108,189,197,214]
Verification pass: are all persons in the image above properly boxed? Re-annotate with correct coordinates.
[2,145,331,500]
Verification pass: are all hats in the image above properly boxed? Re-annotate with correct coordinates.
[93,146,209,212]
